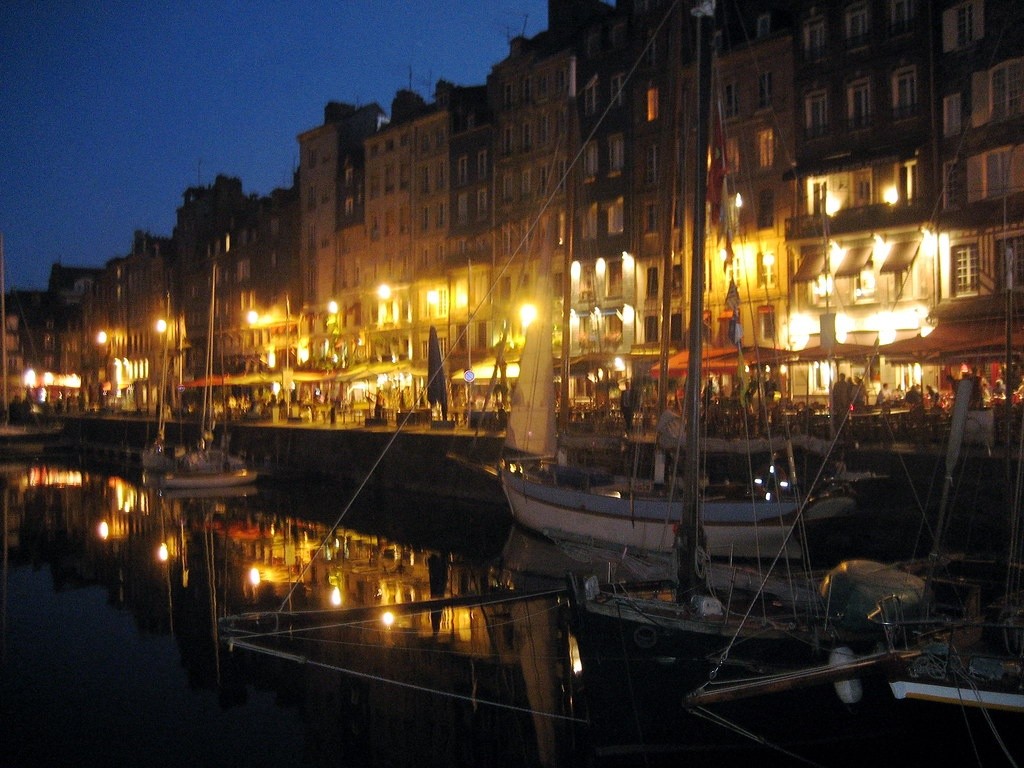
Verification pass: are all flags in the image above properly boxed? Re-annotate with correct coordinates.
[730,306,743,345]
[706,92,740,311]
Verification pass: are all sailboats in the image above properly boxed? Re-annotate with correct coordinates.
[131,1,1024,715]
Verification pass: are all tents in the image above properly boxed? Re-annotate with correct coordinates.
[452,364,522,428]
[186,374,225,419]
[269,373,324,418]
[339,364,406,405]
[555,321,1024,374]
[227,376,268,415]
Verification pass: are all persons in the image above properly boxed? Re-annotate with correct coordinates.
[968,365,984,407]
[182,374,1024,435]
[1010,364,1022,389]
